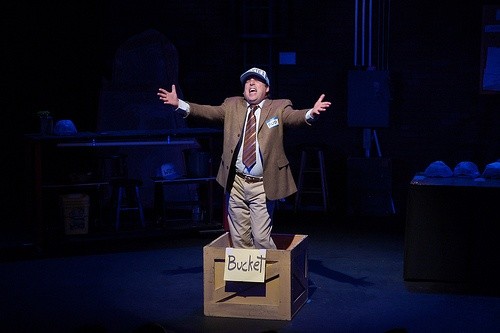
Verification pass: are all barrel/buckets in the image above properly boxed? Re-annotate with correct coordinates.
[63,195,90,234]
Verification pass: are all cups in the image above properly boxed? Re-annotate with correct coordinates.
[40,117,53,136]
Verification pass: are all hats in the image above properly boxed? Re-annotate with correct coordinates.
[454,162,479,176]
[241,67,270,86]
[56,120,76,135]
[482,162,500,176]
[424,161,453,176]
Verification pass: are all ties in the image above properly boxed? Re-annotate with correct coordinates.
[243,105,256,168]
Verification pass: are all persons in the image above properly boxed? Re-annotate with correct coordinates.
[156,67,332,249]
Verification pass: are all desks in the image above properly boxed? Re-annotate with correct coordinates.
[403,174,500,282]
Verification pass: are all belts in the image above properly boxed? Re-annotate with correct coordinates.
[236,172,263,183]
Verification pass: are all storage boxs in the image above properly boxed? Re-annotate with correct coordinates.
[202,230,309,321]
